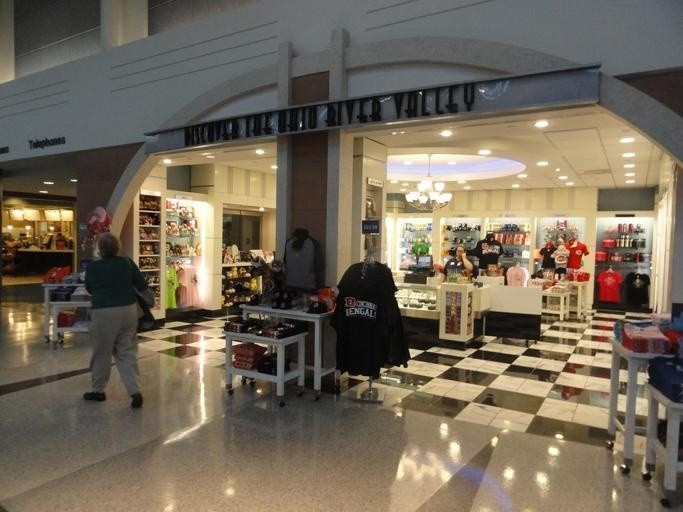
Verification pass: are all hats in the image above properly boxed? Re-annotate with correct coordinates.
[546,243,554,252]
[292,228,308,247]
[557,239,565,245]
[486,234,496,245]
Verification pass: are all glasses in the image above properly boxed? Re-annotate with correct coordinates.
[457,249,463,253]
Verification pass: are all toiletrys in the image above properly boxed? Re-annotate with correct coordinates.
[625,235,629,247]
[618,224,622,233]
[620,235,624,247]
[630,240,632,247]
[623,223,627,234]
[628,224,633,234]
[616,239,619,247]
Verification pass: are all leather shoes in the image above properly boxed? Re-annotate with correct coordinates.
[131,394,143,407]
[83,392,105,402]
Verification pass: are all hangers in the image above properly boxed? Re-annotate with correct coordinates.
[606,266,614,272]
[633,267,643,275]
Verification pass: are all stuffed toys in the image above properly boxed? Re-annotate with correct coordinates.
[87,206,112,235]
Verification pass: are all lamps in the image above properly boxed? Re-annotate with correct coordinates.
[406,154,451,212]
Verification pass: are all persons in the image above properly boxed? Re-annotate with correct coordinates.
[83,233,155,408]
[551,244,570,280]
[476,232,503,269]
[566,238,589,273]
[539,242,558,272]
[444,244,474,280]
[506,262,529,287]
[282,229,325,294]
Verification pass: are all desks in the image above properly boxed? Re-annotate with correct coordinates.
[223,330,309,406]
[239,303,342,400]
[642,383,683,506]
[560,281,587,318]
[49,301,91,350]
[542,290,571,321]
[42,284,85,344]
[606,338,676,472]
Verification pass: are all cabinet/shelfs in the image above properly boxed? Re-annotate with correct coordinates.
[395,283,440,320]
[222,263,263,308]
[122,190,165,329]
[400,222,432,271]
[442,223,480,268]
[440,282,475,341]
[488,224,530,267]
